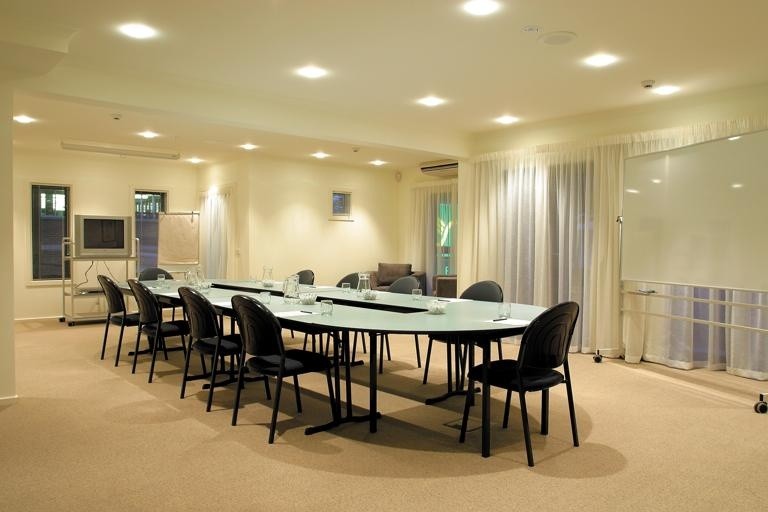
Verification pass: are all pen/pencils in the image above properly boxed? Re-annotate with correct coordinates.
[301,311,312,314]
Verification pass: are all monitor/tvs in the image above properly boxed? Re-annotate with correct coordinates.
[75,215,132,258]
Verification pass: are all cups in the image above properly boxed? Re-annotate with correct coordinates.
[259,292,271,306]
[250,275,257,283]
[157,274,165,282]
[342,283,350,293]
[201,281,211,293]
[411,289,422,304]
[320,300,333,317]
[498,303,511,318]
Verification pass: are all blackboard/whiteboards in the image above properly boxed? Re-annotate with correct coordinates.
[618,128,768,292]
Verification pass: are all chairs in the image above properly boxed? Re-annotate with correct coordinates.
[382,276,424,370]
[173,289,228,414]
[227,294,334,436]
[285,268,315,285]
[97,276,133,371]
[326,271,369,358]
[127,285,184,388]
[461,310,580,468]
[430,280,506,386]
[138,268,173,284]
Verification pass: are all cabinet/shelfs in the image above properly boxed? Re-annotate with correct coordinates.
[62,238,140,322]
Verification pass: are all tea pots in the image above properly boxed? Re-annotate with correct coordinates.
[357,274,371,296]
[282,275,300,304]
[183,266,203,289]
[260,266,274,287]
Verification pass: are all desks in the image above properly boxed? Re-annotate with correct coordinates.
[104,276,552,436]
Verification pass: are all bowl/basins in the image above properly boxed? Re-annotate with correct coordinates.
[299,293,317,305]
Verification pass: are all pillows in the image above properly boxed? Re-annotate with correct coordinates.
[379,264,412,286]
